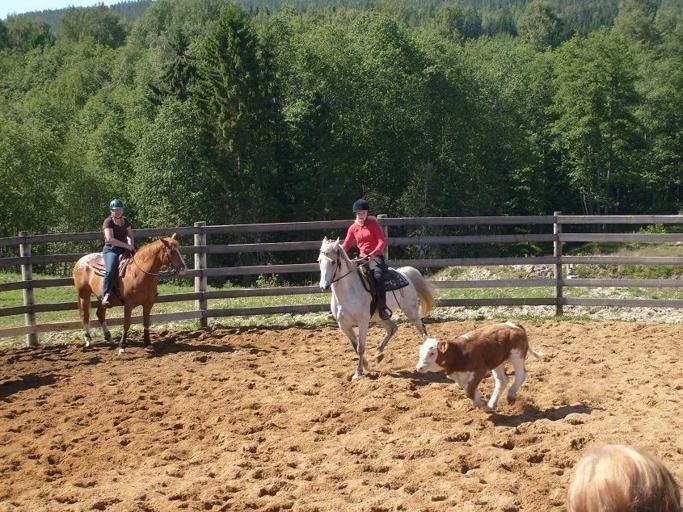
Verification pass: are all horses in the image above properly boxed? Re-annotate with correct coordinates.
[317,236,437,380]
[72,232,187,353]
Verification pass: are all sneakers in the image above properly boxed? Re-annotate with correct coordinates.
[382,311,389,319]
[101,293,112,308]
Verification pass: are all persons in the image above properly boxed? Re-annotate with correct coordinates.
[566,443,682,511]
[339,198,392,320]
[100,199,139,308]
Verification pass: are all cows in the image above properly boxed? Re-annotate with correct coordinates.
[415,321,544,412]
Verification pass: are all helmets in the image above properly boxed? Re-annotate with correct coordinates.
[110,199,124,209]
[353,199,369,212]
